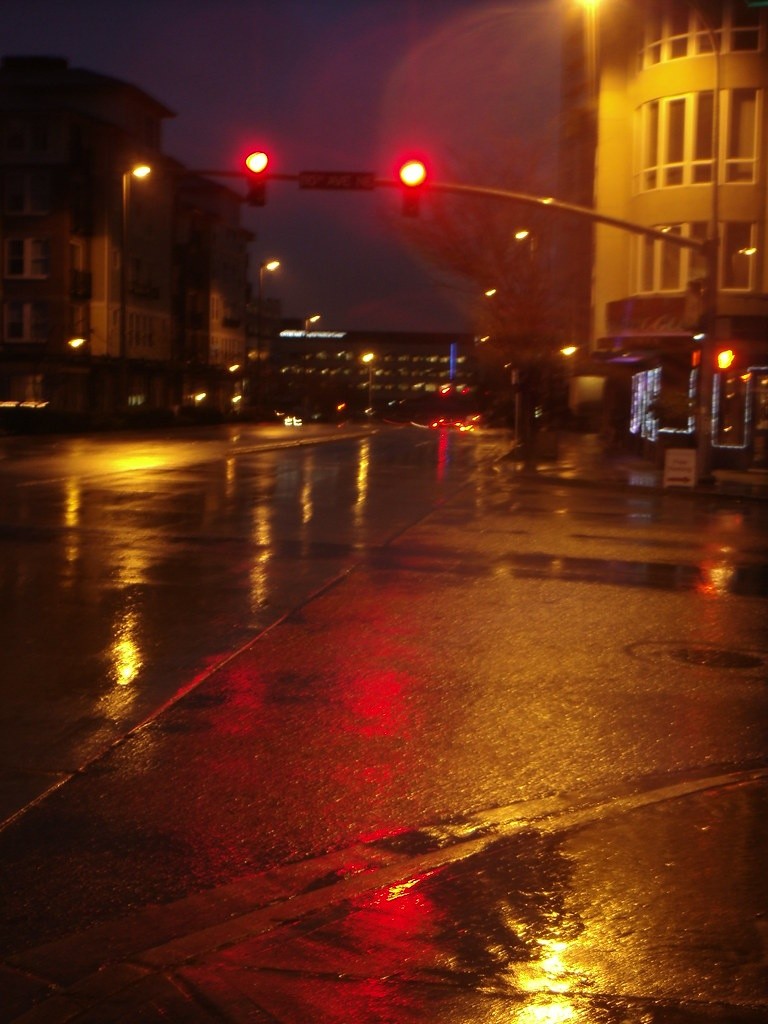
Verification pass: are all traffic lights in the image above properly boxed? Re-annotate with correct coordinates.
[243,149,269,207]
[714,347,740,372]
[399,159,427,217]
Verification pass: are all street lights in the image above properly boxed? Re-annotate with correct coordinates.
[256,256,280,368]
[360,352,375,421]
[119,163,153,355]
[304,314,321,396]
